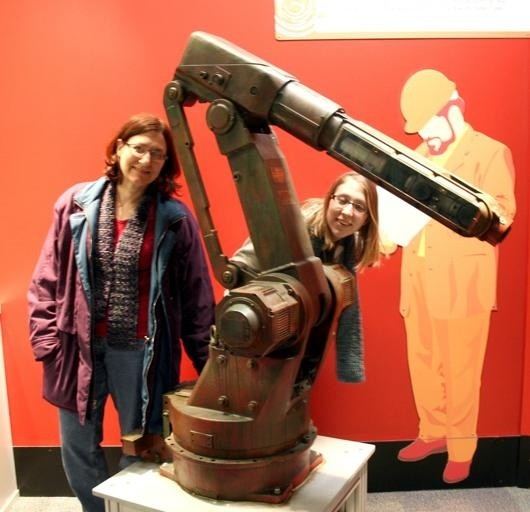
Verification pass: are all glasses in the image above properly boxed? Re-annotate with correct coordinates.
[331,193,369,212]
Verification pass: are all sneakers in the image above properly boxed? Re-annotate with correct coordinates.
[396,437,448,463]
[443,460,472,484]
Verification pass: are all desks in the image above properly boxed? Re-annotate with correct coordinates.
[91,434,378,511]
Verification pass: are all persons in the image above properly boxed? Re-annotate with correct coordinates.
[25,114,216,511]
[225,170,380,382]
[395,70,516,482]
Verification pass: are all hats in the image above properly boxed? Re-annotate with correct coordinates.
[400,69,456,134]
